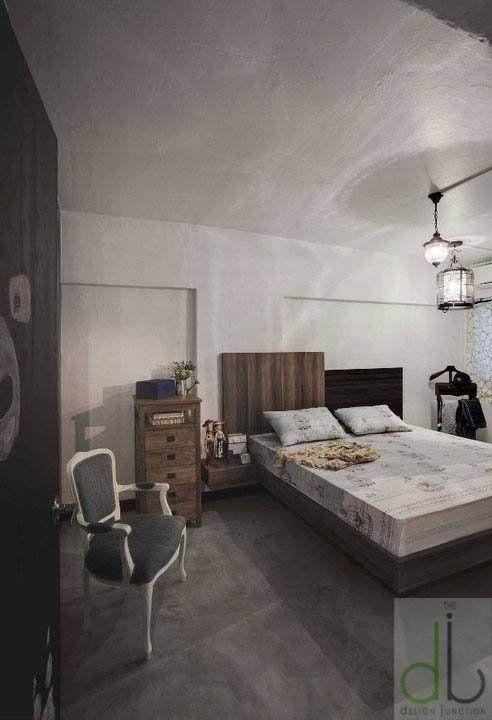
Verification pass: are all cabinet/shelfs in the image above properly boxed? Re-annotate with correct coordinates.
[130,393,203,529]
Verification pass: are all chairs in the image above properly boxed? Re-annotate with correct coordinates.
[66,448,190,659]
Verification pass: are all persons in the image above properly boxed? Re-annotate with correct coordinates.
[214,420,226,459]
[203,418,215,464]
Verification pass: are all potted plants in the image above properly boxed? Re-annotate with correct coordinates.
[172,362,199,396]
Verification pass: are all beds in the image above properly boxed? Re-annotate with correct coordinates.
[221,353,491,597]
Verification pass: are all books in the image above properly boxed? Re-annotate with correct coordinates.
[149,412,184,426]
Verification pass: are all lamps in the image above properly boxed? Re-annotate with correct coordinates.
[437,240,475,311]
[423,191,449,268]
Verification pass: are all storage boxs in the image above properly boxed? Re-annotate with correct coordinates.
[135,379,176,400]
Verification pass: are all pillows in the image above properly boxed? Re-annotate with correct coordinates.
[333,403,413,437]
[259,407,346,448]
